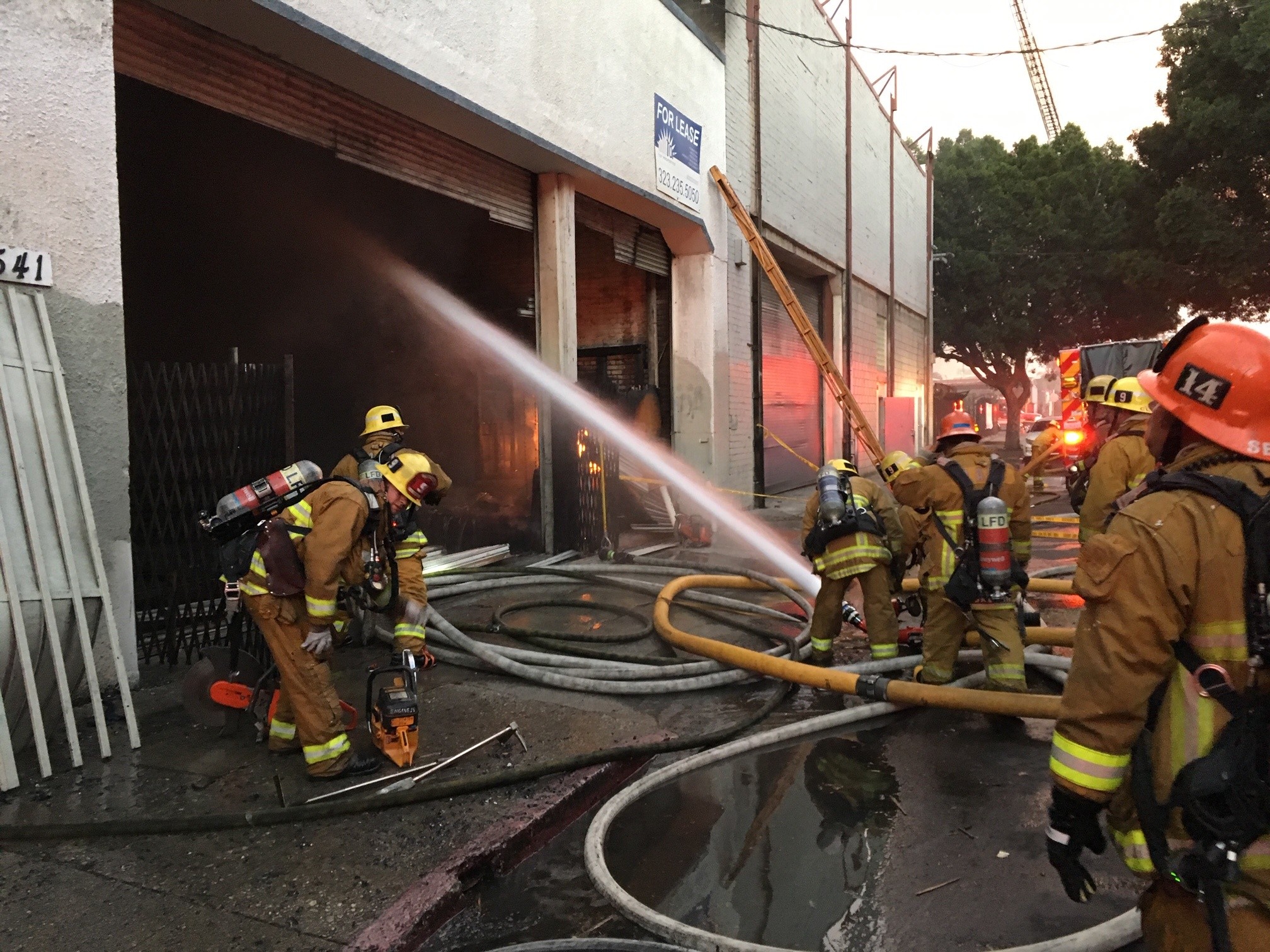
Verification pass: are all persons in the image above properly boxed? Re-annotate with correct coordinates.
[1077,375,1158,547]
[880,412,1032,690]
[216,454,438,780]
[801,459,904,664]
[1046,314,1270,952]
[330,405,451,669]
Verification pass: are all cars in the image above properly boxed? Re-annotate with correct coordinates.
[1024,419,1065,468]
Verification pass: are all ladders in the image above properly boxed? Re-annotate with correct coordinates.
[709,165,892,494]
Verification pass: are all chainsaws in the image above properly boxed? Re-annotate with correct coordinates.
[181,645,357,744]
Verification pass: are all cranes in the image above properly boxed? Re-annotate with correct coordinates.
[1008,1,1063,146]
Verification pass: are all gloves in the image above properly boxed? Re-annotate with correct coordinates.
[423,490,443,507]
[916,441,940,465]
[1046,786,1106,904]
[889,554,908,583]
[300,628,332,655]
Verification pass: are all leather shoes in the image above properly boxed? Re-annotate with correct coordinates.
[270,747,303,757]
[308,756,382,781]
[394,654,438,669]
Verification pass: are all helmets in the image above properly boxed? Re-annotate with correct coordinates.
[1137,316,1270,463]
[826,459,861,477]
[1048,420,1060,430]
[375,453,437,508]
[881,451,921,484]
[1100,376,1160,415]
[936,412,982,442]
[359,405,409,438]
[1081,375,1121,403]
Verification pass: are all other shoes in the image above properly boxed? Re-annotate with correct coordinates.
[916,670,922,683]
